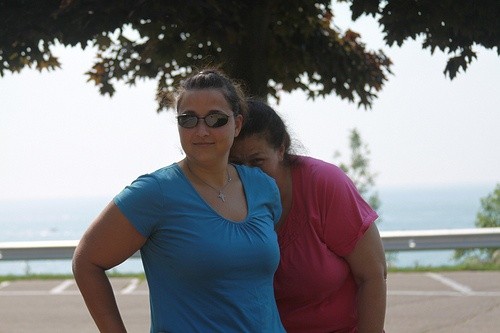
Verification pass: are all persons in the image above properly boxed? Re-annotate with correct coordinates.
[230,97,389,333]
[71,69,287,333]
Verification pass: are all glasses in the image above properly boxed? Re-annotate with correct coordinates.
[175,113,238,129]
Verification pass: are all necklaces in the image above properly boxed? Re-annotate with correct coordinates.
[186,161,228,201]
